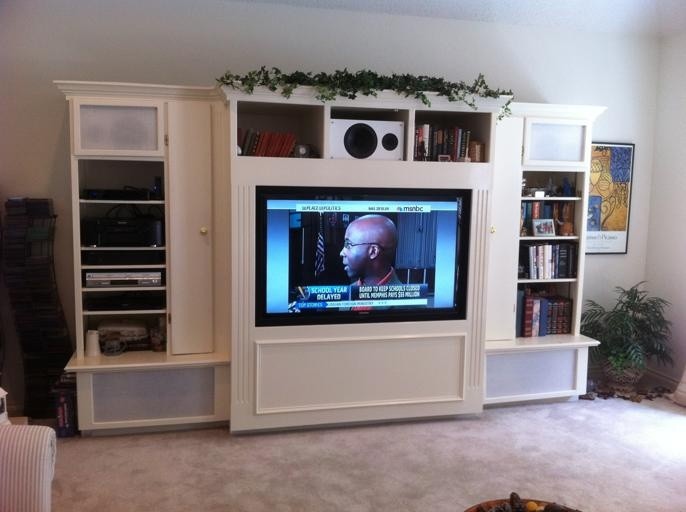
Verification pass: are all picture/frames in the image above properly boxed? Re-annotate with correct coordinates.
[587,137,635,258]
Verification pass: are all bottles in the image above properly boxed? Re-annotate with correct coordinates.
[55,391,72,438]
[294,143,311,158]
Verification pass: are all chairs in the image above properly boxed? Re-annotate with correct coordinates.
[0,421,60,512]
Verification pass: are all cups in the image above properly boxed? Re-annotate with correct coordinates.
[102,330,128,356]
[85,329,101,357]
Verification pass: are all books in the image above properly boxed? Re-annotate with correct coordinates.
[515,200,580,339]
[412,120,488,165]
[0,195,80,437]
[232,126,302,156]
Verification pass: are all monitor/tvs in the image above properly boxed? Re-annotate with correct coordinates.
[255,185,472,326]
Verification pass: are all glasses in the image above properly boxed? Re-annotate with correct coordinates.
[344,240,385,249]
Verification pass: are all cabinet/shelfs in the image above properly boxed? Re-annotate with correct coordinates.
[51,78,233,437]
[479,98,612,408]
[215,69,514,188]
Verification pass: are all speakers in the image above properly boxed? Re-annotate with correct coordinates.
[328,117,405,161]
[82,215,163,246]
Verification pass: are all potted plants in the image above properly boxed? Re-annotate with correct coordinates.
[578,278,678,403]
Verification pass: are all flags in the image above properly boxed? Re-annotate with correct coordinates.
[312,213,328,282]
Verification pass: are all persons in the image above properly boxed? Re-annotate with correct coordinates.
[339,213,427,310]
